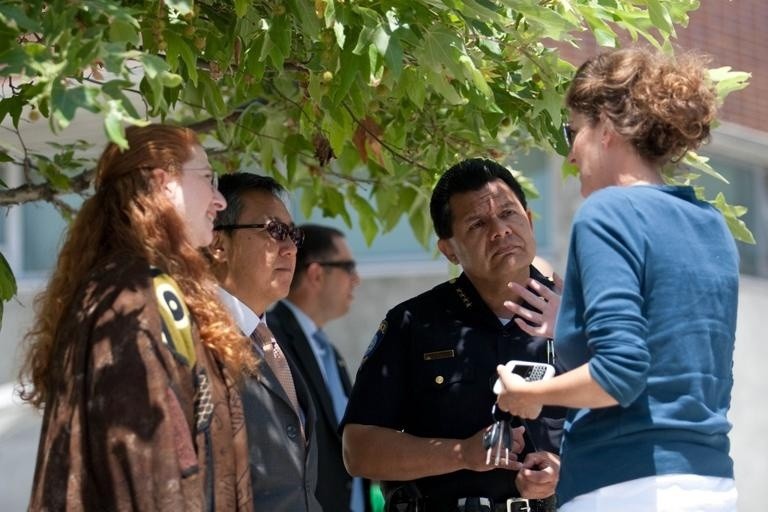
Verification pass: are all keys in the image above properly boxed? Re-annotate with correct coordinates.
[494,419,505,467]
[481,423,499,466]
[501,421,512,467]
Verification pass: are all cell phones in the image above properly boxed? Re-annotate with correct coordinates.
[492,359,556,397]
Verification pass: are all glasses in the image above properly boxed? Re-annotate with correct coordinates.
[173,168,219,192]
[564,122,574,146]
[319,261,355,271]
[214,221,304,248]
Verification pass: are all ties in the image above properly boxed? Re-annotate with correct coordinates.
[252,322,306,440]
[311,330,365,512]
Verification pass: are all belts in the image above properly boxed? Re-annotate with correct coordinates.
[494,498,556,511]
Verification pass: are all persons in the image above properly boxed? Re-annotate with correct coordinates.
[264,221,372,512]
[10,121,262,512]
[493,45,740,512]
[335,156,565,511]
[194,170,326,512]
